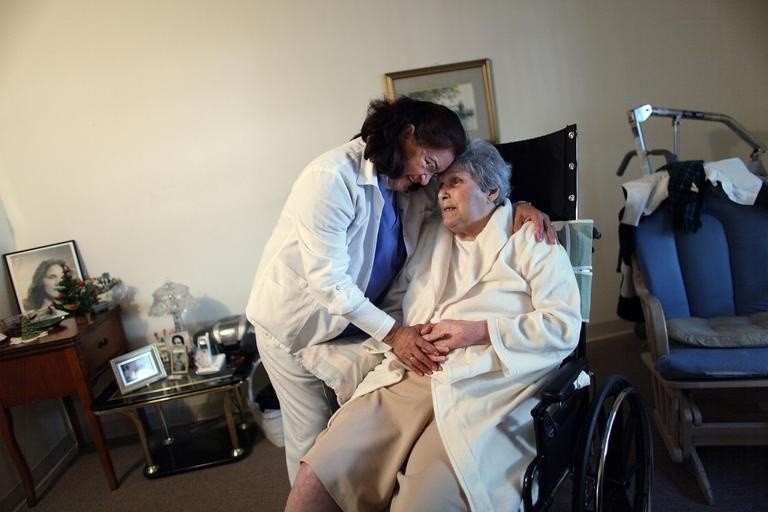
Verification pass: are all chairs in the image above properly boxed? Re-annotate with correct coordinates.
[620,159,764,506]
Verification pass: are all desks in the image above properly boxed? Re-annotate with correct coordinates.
[2,305,152,510]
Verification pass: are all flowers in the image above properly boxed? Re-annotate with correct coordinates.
[58,264,121,314]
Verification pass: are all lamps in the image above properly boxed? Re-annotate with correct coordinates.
[149,280,201,354]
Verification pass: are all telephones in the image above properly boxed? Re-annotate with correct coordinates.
[194,332,226,377]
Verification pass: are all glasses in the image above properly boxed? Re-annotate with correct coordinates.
[418,138,440,178]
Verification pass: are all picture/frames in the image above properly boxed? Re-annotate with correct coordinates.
[383,56,499,144]
[6,237,89,323]
[111,343,190,395]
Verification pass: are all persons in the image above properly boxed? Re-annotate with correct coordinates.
[283,140,579,510]
[172,335,185,371]
[246,98,559,489]
[22,259,66,311]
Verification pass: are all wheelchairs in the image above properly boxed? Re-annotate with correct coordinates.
[520,222,661,512]
[612,104,767,504]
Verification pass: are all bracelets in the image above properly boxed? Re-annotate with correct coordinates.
[514,200,528,206]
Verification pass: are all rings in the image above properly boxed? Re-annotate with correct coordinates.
[550,224,553,227]
[409,357,414,362]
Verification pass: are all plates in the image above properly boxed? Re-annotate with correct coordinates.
[30,316,64,331]
